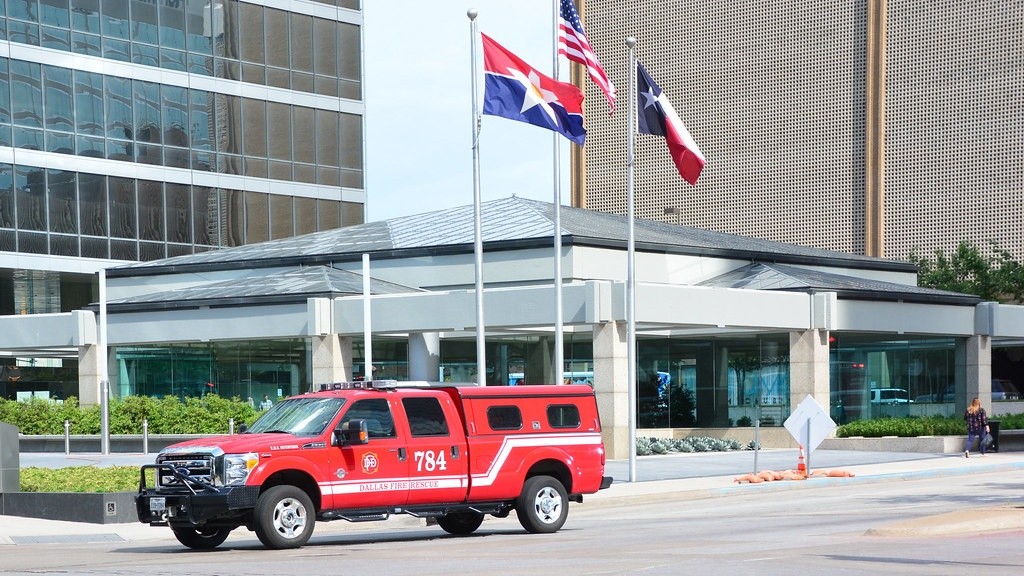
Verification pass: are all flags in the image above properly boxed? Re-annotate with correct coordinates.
[479,31,587,149]
[558,0,618,117]
[634,60,706,186]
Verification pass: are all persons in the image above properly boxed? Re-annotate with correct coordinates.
[963,398,990,457]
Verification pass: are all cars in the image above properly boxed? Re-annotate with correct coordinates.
[915,378,1024,401]
[871,388,914,405]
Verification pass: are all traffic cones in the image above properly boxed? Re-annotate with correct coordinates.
[797,445,806,476]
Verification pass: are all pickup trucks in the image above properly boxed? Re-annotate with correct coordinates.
[134,378,614,551]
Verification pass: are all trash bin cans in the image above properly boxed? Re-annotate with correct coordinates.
[978,421,1000,453]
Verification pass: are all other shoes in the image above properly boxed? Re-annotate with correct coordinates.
[981,455,987,457]
[965,450,969,458]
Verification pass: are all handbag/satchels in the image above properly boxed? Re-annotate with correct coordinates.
[980,433,993,447]
